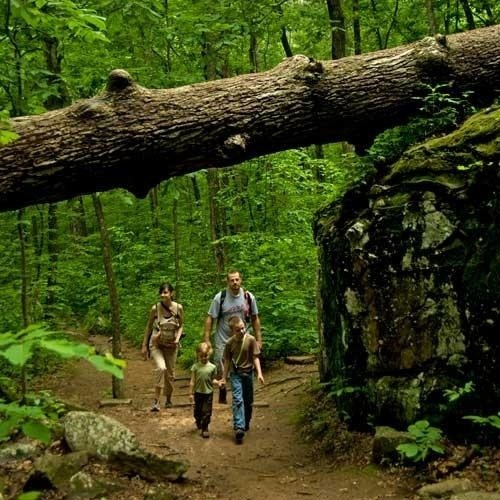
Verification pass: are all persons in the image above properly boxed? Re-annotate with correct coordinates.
[139,281,185,413]
[217,316,266,445]
[189,341,227,438]
[202,269,262,405]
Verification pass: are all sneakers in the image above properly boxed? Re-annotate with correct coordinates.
[165,402,171,407]
[219,387,227,404]
[195,419,202,429]
[151,398,160,410]
[234,429,244,443]
[202,430,209,438]
[245,426,250,431]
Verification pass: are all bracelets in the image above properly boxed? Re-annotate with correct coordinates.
[141,343,148,347]
